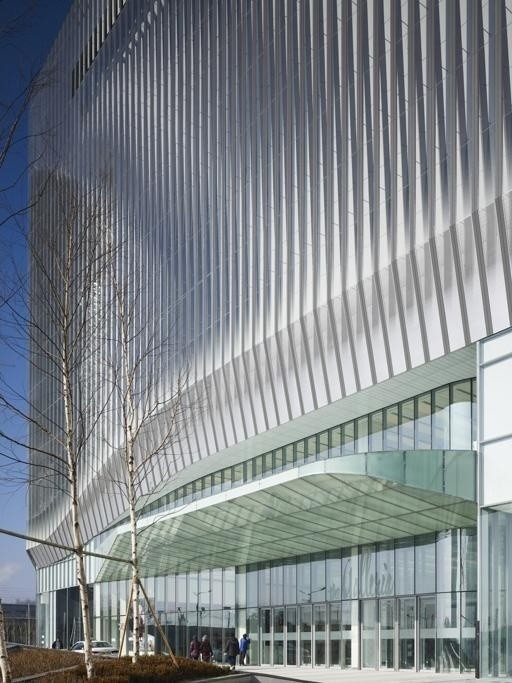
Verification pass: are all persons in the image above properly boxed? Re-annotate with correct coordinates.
[199,634,213,663]
[224,632,239,671]
[189,634,201,661]
[52,636,63,649]
[239,633,250,666]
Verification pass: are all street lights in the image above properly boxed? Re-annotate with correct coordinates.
[192,590,212,627]
[299,587,326,603]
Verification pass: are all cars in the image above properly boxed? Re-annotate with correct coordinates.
[69,641,119,657]
